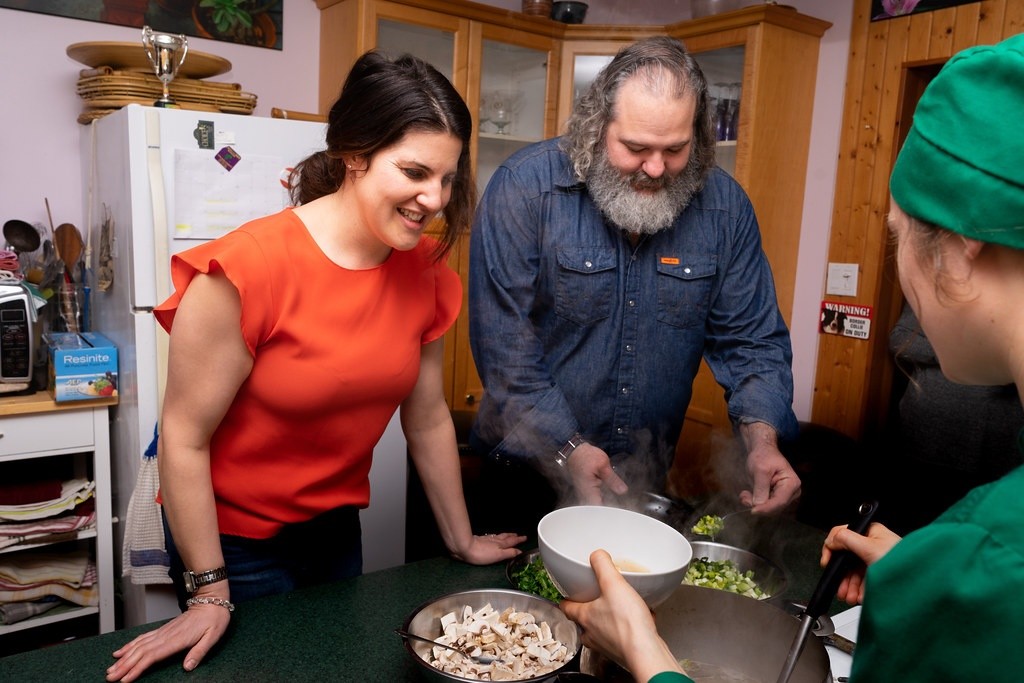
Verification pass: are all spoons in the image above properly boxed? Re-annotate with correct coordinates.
[696,508,753,535]
[393,628,505,662]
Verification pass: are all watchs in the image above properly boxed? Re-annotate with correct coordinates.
[183,567,228,593]
[552,433,585,472]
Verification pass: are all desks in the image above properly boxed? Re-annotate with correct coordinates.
[0,515,856,683]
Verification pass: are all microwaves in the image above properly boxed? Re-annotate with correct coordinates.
[0,285,32,394]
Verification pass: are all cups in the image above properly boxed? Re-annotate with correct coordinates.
[551,671,601,683]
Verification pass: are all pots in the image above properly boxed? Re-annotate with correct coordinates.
[557,585,835,683]
[402,589,582,683]
[505,541,788,610]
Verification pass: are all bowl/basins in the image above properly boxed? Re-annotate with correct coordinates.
[521,0,588,24]
[537,506,694,610]
[690,0,741,19]
[639,490,694,533]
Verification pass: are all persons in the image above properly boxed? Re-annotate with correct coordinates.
[106,52,526,683]
[556,29,1024,683]
[466,35,803,553]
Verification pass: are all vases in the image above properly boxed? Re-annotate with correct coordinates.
[552,1,589,24]
[521,0,554,18]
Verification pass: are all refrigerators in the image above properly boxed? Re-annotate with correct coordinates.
[82,103,406,626]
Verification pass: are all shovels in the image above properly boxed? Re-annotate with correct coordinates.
[53,224,83,275]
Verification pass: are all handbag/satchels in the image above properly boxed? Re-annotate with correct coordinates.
[119,420,174,583]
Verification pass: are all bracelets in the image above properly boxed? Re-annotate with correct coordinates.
[187,596,235,612]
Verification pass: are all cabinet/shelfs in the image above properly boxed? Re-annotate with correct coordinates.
[0,360,121,653]
[313,0,834,502]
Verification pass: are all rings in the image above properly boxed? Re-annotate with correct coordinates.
[485,534,487,535]
[491,534,496,537]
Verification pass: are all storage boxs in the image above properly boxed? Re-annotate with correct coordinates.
[41,331,119,402]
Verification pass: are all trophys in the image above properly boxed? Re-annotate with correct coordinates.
[142,25,188,109]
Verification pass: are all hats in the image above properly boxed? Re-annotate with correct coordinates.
[890,31,1024,251]
